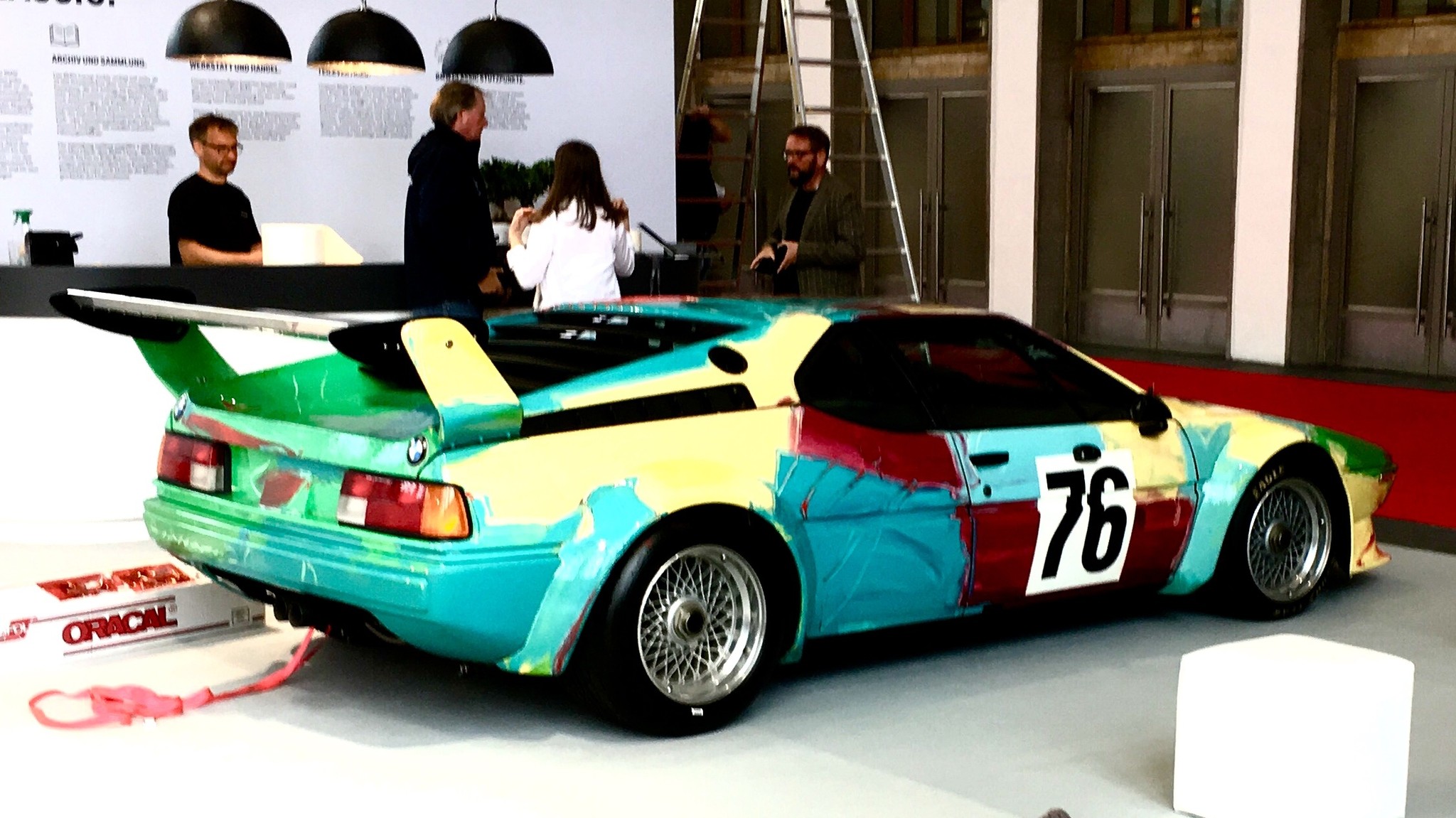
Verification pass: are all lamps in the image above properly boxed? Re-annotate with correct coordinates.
[164,0,292,61]
[441,0,554,75]
[307,0,425,73]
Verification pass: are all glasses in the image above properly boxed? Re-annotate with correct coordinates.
[200,140,243,157]
[784,149,820,162]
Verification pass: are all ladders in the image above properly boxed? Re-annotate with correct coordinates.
[675,0,919,299]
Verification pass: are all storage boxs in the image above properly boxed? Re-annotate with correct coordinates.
[0,563,267,664]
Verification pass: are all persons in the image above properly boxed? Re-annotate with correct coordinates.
[168,116,263,266]
[507,139,634,309]
[749,125,868,297]
[404,81,504,315]
[676,107,732,242]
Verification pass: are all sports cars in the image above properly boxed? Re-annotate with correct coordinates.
[49,286,1396,737]
